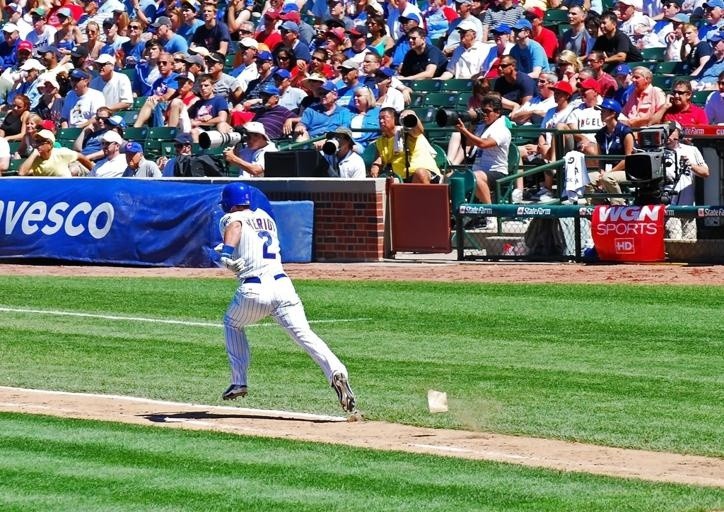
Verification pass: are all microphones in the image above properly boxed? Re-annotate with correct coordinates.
[674,138,685,143]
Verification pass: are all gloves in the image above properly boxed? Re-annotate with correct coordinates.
[220,256,246,273]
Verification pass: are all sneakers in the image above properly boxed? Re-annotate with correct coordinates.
[222,385,249,400]
[333,370,355,415]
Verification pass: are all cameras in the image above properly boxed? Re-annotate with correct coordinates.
[199,130,248,149]
[323,138,339,155]
[436,107,484,127]
[399,114,417,128]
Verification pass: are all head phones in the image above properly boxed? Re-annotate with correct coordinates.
[676,120,685,138]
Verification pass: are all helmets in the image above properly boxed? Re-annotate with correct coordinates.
[218,182,252,213]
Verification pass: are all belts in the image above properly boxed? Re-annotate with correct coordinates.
[243,273,287,283]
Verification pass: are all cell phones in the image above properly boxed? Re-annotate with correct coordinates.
[671,92,674,102]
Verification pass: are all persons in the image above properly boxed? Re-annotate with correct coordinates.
[0,0,722,261]
[199,181,355,415]
